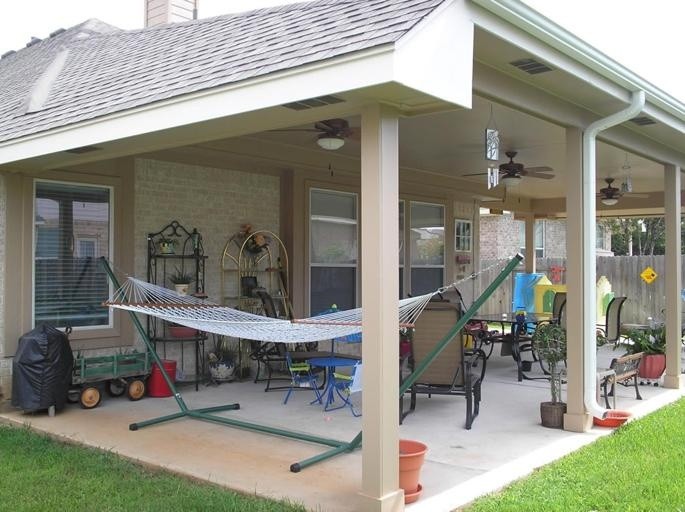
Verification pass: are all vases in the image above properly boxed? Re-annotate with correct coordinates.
[399,439,427,503]
[243,276,257,297]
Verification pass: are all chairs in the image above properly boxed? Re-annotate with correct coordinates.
[439,287,493,359]
[399,303,486,429]
[284,352,322,405]
[252,291,339,391]
[552,291,566,317]
[597,296,627,351]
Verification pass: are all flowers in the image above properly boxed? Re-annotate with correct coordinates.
[239,224,269,253]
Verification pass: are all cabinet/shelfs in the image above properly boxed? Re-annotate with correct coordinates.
[221,231,289,381]
[146,218,208,391]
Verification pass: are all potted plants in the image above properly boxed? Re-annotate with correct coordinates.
[208,346,236,378]
[533,325,567,428]
[169,273,191,295]
[163,319,197,337]
[158,238,178,255]
[614,329,666,378]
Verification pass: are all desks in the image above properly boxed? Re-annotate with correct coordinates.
[471,312,559,383]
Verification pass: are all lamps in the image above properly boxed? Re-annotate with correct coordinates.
[486,104,499,190]
[502,173,522,185]
[601,195,619,205]
[317,132,345,150]
[622,152,632,193]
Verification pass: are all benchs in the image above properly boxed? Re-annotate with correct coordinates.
[601,352,644,408]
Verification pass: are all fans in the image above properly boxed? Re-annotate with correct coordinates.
[267,119,361,135]
[461,152,555,179]
[596,177,648,198]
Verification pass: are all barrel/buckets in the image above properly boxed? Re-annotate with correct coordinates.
[512,273,543,333]
[147,359,177,398]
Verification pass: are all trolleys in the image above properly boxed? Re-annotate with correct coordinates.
[60,323,152,411]
